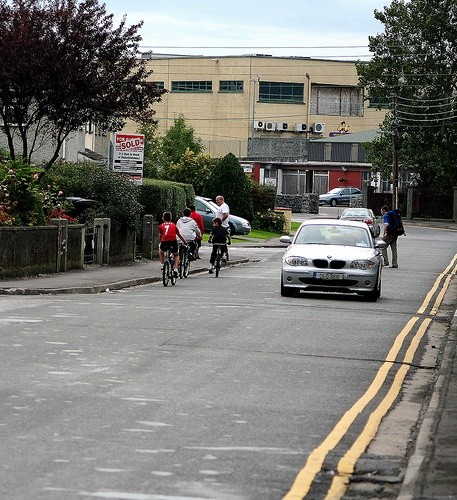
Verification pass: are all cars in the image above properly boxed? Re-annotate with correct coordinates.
[318,187,363,207]
[337,207,381,239]
[279,217,387,302]
[194,195,252,237]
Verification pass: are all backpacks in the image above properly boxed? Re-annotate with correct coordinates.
[387,211,404,237]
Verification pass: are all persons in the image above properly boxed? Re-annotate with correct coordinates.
[188,204,204,259]
[175,208,202,261]
[214,196,230,230]
[381,206,398,268]
[207,217,231,274]
[158,211,188,278]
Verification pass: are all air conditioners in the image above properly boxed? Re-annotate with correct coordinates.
[295,124,307,131]
[254,121,265,129]
[266,122,276,131]
[313,123,325,132]
[277,122,289,131]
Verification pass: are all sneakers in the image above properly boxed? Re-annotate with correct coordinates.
[173,268,179,278]
[187,254,196,261]
[161,264,164,270]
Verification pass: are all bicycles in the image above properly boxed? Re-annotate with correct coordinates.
[176,235,202,279]
[208,241,230,277]
[161,242,187,286]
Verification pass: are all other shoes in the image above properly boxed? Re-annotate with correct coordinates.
[223,253,227,261]
[210,264,215,270]
[382,264,389,266]
[389,266,398,268]
